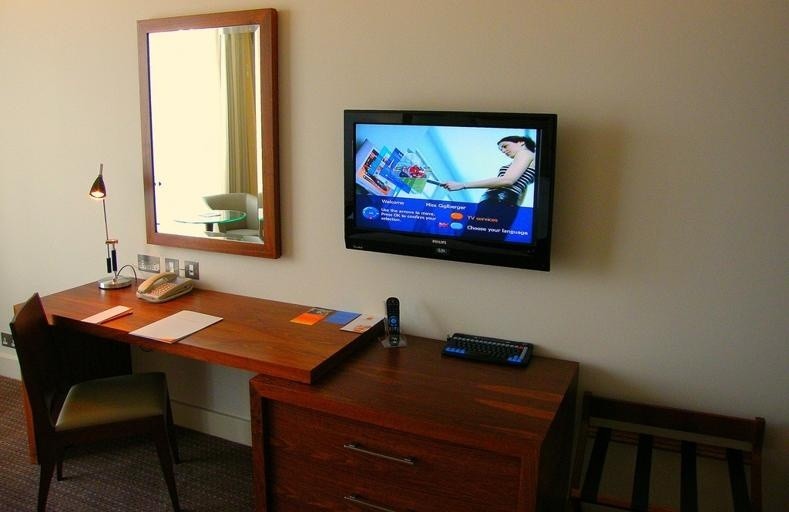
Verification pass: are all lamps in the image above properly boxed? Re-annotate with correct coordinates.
[90,163,132,290]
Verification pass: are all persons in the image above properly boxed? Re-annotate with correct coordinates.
[445,136,536,242]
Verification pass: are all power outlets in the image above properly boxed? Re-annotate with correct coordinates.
[165,258,178,275]
[185,261,200,280]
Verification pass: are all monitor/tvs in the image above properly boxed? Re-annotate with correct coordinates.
[342,108,558,272]
[355,137,401,198]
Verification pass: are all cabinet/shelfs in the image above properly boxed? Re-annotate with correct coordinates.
[568,393,767,511]
[247,326,579,511]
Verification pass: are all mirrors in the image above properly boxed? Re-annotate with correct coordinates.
[137,9,282,259]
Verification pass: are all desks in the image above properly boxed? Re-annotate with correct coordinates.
[173,210,246,231]
[13,276,385,465]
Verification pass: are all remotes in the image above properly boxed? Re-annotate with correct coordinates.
[426,178,447,188]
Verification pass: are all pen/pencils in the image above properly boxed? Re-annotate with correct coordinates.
[99,310,133,324]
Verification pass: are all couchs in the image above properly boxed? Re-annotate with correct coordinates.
[198,193,259,236]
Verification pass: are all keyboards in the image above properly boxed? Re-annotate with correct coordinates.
[441,332,535,369]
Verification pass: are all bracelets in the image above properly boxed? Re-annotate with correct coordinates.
[464,183,466,189]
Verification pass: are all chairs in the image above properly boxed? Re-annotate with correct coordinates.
[9,293,181,512]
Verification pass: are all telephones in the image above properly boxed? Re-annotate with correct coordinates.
[136,272,194,303]
